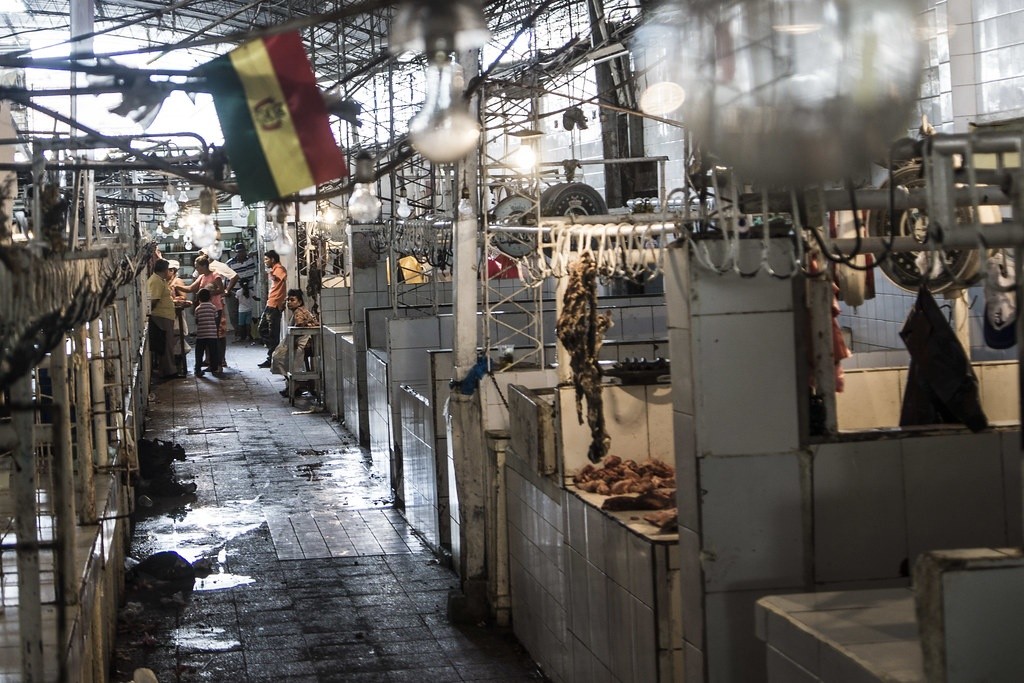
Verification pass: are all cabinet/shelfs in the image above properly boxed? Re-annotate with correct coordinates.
[286,325,322,407]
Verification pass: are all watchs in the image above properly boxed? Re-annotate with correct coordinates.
[224,289,228,294]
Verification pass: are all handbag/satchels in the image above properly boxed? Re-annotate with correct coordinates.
[173,314,188,337]
[173,337,191,356]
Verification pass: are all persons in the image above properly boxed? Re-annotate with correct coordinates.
[270,289,320,396]
[235,277,253,342]
[169,250,239,372]
[223,243,258,341]
[257,250,287,367]
[146,259,191,379]
[194,288,223,378]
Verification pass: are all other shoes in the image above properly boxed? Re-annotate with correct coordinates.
[203,367,211,372]
[221,360,227,367]
[201,361,208,366]
[258,361,271,368]
[212,370,219,376]
[279,388,288,396]
[194,371,202,377]
[168,373,186,378]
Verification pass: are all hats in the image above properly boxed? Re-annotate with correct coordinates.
[166,260,180,270]
[235,243,246,252]
[198,248,209,257]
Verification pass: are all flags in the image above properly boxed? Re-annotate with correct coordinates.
[204,31,348,206]
